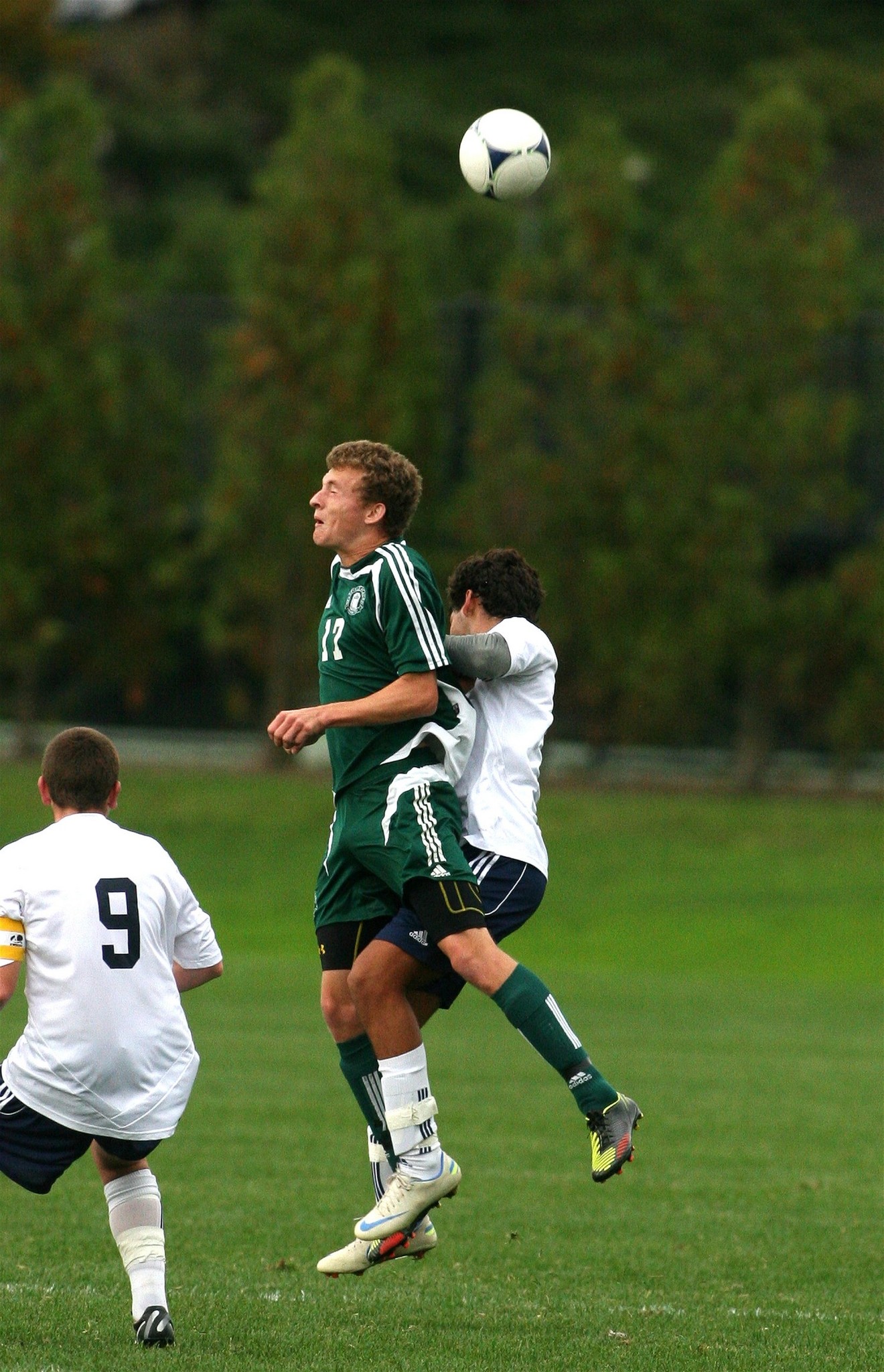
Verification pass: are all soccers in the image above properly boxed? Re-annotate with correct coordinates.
[459,108,552,202]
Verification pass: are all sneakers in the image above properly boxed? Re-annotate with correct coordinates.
[317,1212,437,1278]
[367,1185,458,1264]
[354,1150,462,1241]
[584,1092,644,1184]
[133,1305,174,1348]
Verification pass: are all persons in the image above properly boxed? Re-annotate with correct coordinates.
[314,548,557,1276]
[269,438,641,1264]
[0,724,224,1349]
